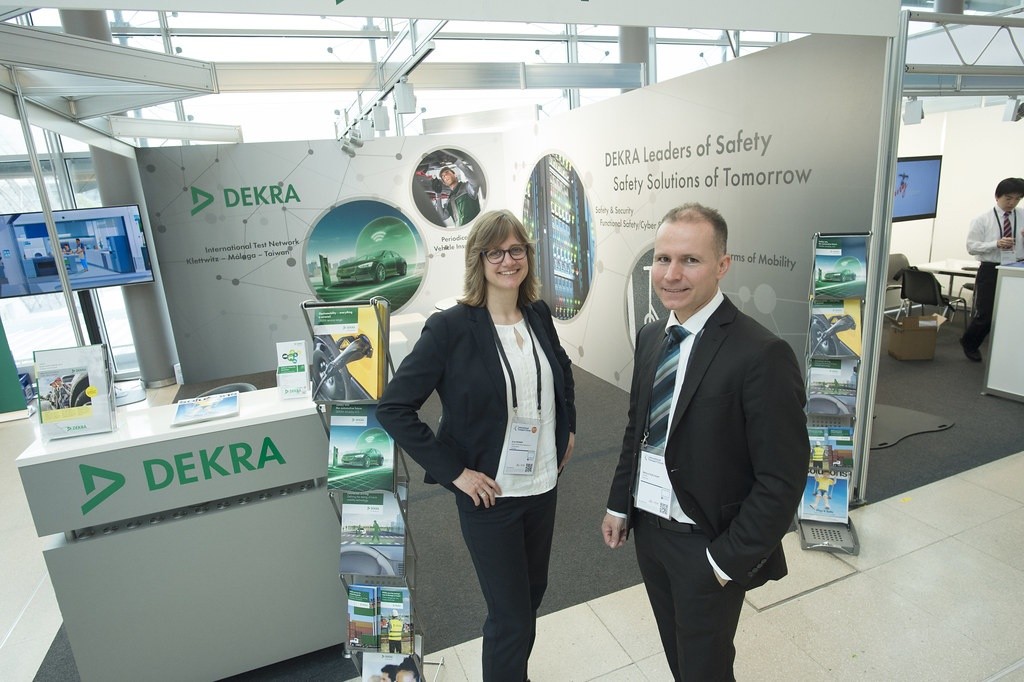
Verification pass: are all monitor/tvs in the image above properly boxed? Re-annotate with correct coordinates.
[0,204,155,299]
[891,155,943,222]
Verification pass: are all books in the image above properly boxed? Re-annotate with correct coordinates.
[338,475,409,577]
[345,585,415,654]
[805,352,858,417]
[309,302,387,405]
[326,404,398,495]
[362,633,424,682]
[811,234,869,300]
[171,390,240,427]
[33,343,116,441]
[797,426,855,524]
[809,298,862,359]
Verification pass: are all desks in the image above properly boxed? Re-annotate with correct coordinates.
[15,382,350,682]
[937,270,977,295]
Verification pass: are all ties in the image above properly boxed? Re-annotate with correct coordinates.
[648,326,692,455]
[1003,212,1012,251]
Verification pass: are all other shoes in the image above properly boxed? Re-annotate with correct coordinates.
[959,338,982,363]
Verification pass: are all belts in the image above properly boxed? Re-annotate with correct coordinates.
[638,510,705,533]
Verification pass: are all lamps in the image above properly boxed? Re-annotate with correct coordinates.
[372,102,390,131]
[902,97,924,126]
[348,126,364,148]
[394,77,416,115]
[341,135,356,158]
[1002,95,1024,122]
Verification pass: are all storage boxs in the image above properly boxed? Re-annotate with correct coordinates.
[885,313,948,361]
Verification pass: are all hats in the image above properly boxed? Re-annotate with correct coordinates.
[438,167,455,179]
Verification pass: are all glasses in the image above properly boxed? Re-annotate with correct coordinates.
[482,243,529,264]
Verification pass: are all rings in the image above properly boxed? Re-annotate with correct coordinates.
[481,492,487,497]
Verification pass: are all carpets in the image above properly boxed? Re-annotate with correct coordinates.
[32,360,798,682]
[866,307,1024,502]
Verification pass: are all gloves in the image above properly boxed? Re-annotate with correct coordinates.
[448,155,456,164]
[431,178,442,193]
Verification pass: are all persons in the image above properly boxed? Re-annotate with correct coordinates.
[959,177,1024,362]
[601,201,813,682]
[431,161,480,226]
[374,209,577,682]
[62,238,90,272]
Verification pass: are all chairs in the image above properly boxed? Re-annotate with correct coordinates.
[900,268,966,330]
[950,283,975,322]
[884,253,924,317]
